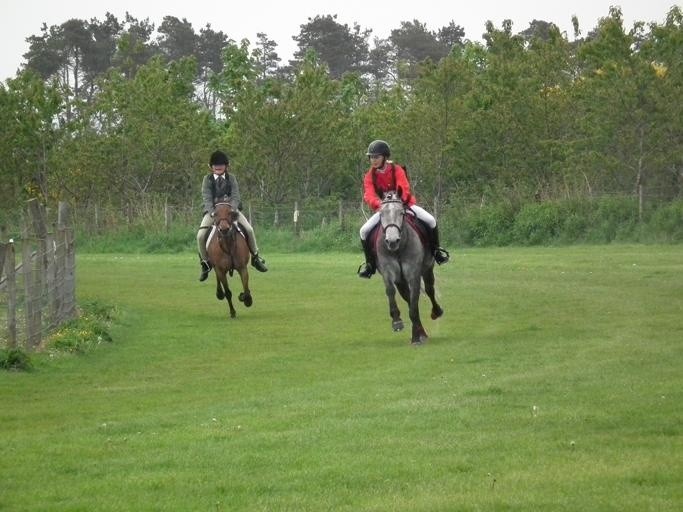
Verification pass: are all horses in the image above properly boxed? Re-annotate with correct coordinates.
[204,194,252,318]
[372,185,443,346]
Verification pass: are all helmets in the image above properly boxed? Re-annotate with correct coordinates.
[365,140,390,155]
[209,151,229,164]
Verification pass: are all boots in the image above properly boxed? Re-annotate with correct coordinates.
[199,260,213,281]
[359,240,372,277]
[431,225,445,264]
[251,253,268,272]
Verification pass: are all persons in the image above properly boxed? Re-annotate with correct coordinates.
[355,141,451,277]
[196,151,268,282]
